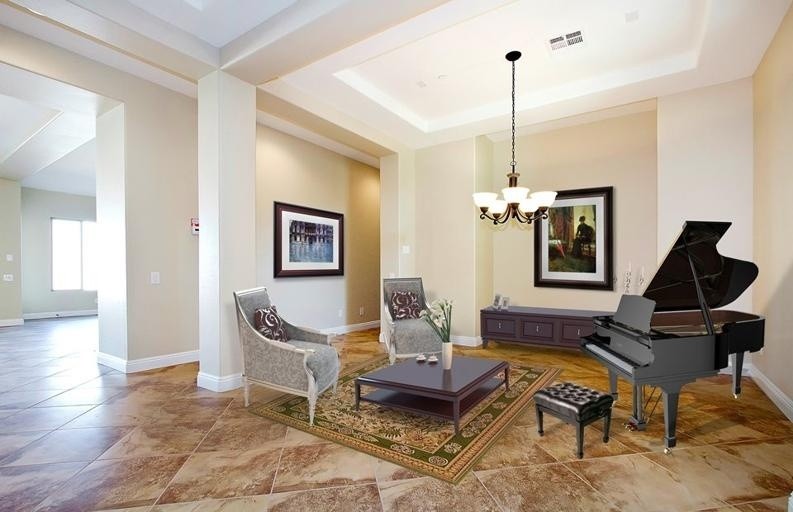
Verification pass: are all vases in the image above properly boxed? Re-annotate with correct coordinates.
[441,342,452,370]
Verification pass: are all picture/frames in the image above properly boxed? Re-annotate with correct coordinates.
[273,201,344,277]
[492,294,502,309]
[502,297,509,310]
[534,187,614,291]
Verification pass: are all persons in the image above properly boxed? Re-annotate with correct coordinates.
[572,216,593,258]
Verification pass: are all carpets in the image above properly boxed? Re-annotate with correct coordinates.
[248,351,564,486]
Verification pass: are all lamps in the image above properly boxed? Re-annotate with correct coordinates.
[471,51,557,225]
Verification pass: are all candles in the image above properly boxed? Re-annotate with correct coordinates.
[628,259,631,272]
[641,265,645,278]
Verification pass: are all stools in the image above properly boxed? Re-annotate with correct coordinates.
[533,381,614,459]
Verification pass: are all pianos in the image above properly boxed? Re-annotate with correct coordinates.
[577,221,764,456]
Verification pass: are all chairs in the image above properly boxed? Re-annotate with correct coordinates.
[381,275,443,365]
[233,287,341,426]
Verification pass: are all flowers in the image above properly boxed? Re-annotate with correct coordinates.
[418,298,453,342]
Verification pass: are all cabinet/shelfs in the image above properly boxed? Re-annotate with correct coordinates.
[480,306,616,349]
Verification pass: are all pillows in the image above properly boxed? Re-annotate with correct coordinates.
[254,305,291,343]
[391,289,422,320]
